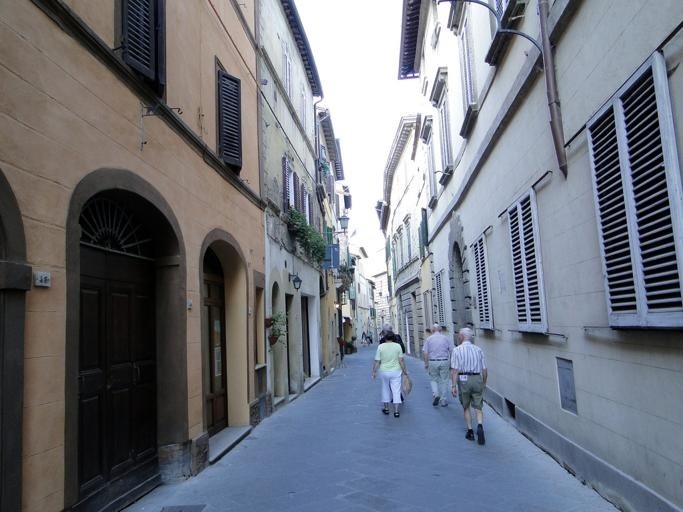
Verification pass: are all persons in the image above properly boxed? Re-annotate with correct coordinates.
[372,332,409,417]
[377,323,405,356]
[420,322,453,409]
[448,326,488,446]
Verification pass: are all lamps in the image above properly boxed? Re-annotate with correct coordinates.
[289,271,302,291]
[333,212,349,238]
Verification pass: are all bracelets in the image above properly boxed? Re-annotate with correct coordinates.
[452,383,456,386]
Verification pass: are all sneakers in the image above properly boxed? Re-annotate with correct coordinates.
[381,407,400,418]
[431,395,449,407]
[465,424,485,445]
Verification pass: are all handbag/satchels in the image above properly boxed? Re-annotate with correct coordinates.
[403,374,412,395]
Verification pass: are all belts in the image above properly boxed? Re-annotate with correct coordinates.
[428,359,448,361]
[458,372,480,375]
